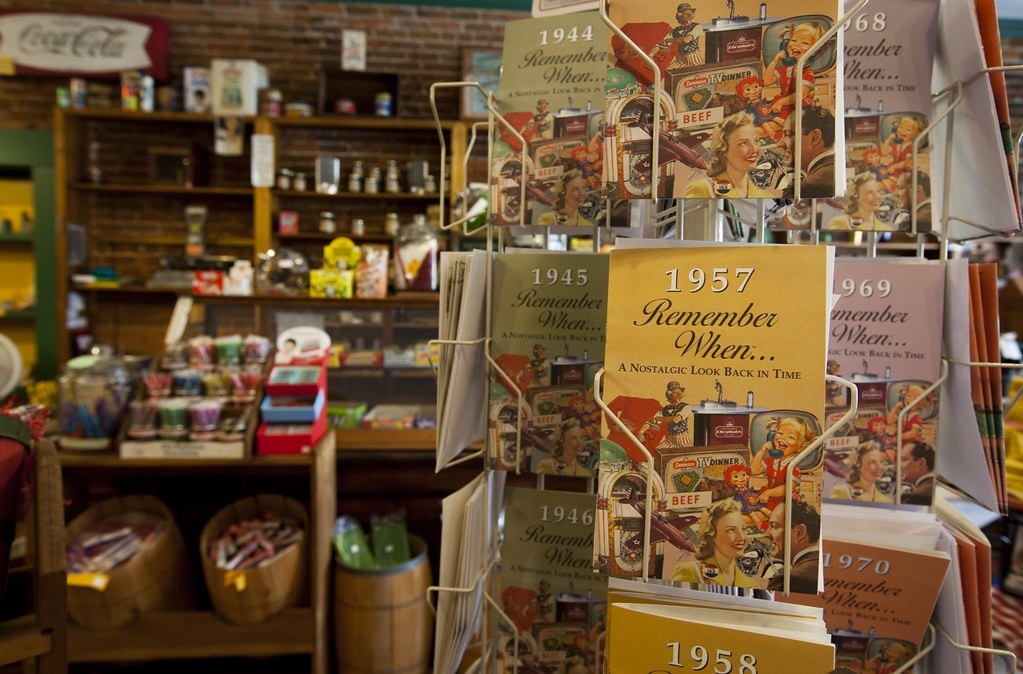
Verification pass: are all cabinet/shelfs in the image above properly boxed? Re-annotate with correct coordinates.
[36,434,334,672]
[1,126,52,418]
[53,94,266,368]
[268,118,461,449]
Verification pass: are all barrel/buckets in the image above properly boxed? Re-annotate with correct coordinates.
[336,534,431,673]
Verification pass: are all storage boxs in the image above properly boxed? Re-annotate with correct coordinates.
[255,351,326,458]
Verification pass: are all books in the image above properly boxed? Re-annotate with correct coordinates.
[417,0,1023,674]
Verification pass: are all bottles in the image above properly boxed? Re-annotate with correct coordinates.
[86,143,102,181]
[747,391,753,408]
[879,101,883,111]
[584,350,587,360]
[760,3,767,20]
[350,159,402,192]
[385,211,401,236]
[320,212,336,231]
[186,225,202,264]
[886,367,890,378]
[588,589,591,601]
[278,168,305,190]
[868,627,874,636]
[352,219,364,236]
[587,101,592,111]
